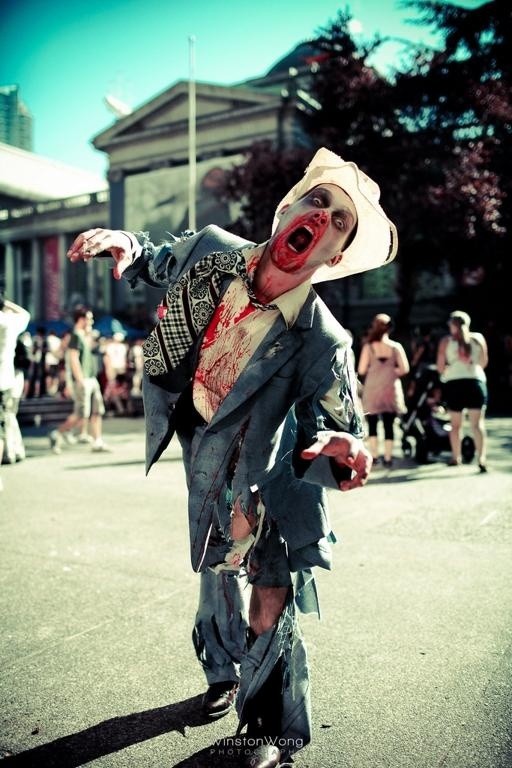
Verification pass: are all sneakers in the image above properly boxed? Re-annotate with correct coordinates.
[448,456,490,472]
[372,458,394,466]
[49,435,114,454]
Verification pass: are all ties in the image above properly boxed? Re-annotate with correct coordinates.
[141,245,279,375]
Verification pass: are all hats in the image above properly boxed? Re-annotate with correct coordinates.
[272,147,398,287]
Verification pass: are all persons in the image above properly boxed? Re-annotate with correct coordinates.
[0,295,146,466]
[344,310,490,472]
[62,145,401,767]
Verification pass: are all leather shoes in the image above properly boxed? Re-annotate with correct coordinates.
[202,683,236,720]
[246,742,282,768]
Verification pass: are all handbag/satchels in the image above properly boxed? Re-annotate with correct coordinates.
[14,341,33,374]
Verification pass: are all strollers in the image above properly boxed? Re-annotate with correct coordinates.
[400,359,475,464]
